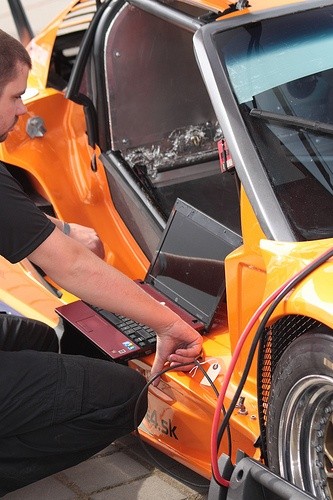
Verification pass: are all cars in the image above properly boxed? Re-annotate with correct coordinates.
[0,0,333,500]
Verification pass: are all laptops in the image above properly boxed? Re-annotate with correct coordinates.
[54,198,244,363]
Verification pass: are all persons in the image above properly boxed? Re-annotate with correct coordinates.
[0,29,206,497]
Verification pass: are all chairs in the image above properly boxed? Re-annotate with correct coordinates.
[94,0,220,170]
